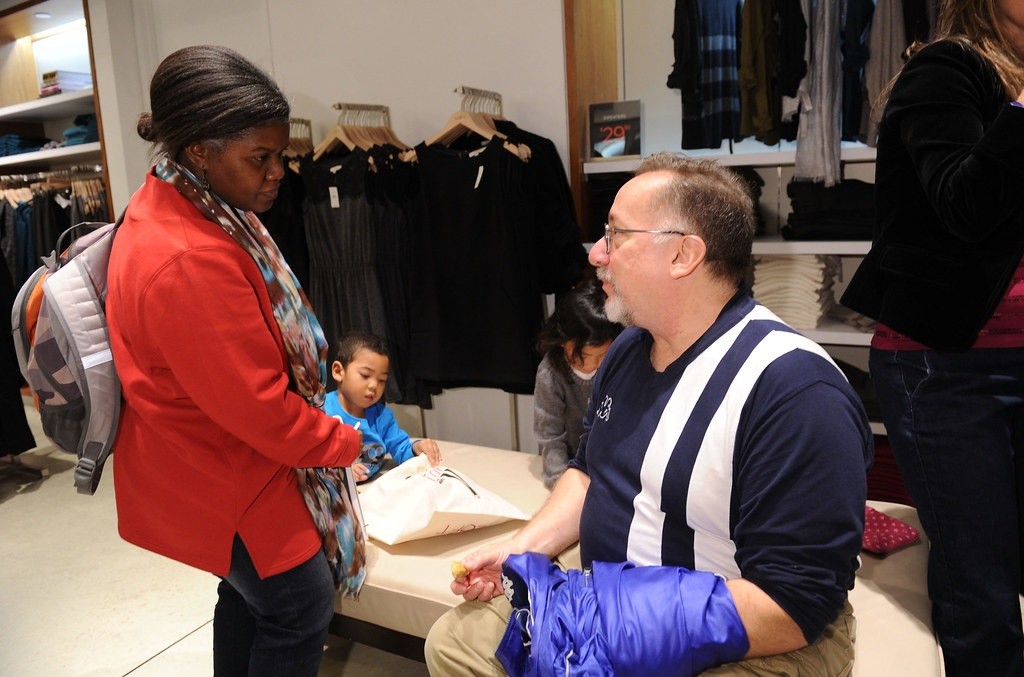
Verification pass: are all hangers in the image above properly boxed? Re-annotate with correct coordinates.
[285,117,313,174]
[303,103,412,173]
[400,86,533,166]
[0,164,105,218]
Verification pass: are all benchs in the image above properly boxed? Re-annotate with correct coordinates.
[333,436,945,677]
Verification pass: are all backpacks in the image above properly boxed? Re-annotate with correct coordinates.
[11,181,148,496]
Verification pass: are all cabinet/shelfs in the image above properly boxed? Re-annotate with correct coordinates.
[0,0,102,166]
[569,147,882,347]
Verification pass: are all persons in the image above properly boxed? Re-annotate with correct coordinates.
[534,274,626,493]
[106,45,366,677]
[425,152,875,677]
[840,0,1024,677]
[317,330,443,483]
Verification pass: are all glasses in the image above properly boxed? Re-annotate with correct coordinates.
[604,224,685,254]
